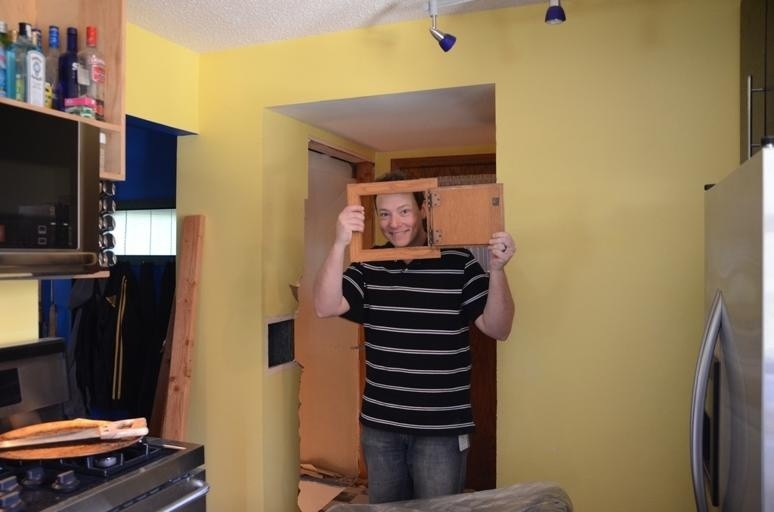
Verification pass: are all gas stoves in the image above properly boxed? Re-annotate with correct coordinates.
[0,443,183,510]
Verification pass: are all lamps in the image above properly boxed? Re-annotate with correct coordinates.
[544,1,566,24]
[425,1,457,52]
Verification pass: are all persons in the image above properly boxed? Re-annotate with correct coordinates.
[314,169,516,504]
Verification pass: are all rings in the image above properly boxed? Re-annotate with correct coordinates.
[502,245,507,252]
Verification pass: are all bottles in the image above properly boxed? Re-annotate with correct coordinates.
[0,20,109,121]
